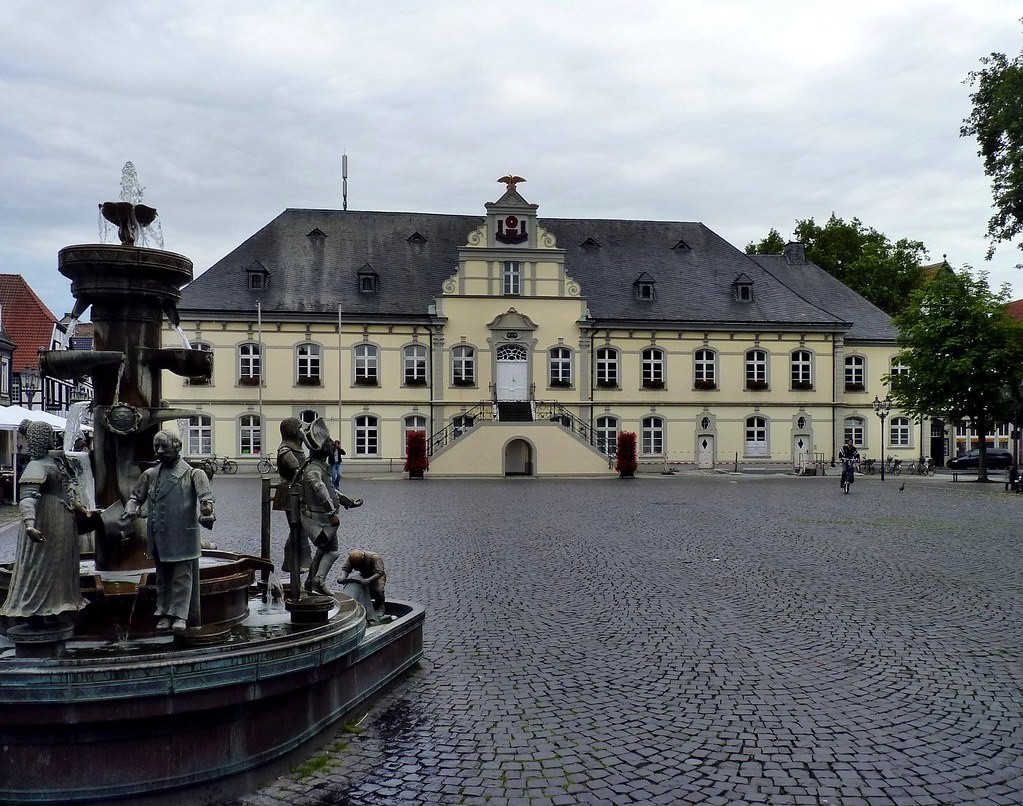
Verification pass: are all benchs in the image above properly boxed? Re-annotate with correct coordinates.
[952,473,1006,482]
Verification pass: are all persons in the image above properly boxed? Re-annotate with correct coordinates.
[839,438,860,488]
[272,417,314,571]
[0,418,101,618]
[328,440,346,491]
[341,549,386,616]
[122,429,216,630]
[298,435,363,596]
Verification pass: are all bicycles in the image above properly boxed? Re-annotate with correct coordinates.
[859,453,877,474]
[908,455,937,476]
[208,453,239,474]
[839,456,859,493]
[257,452,281,473]
[884,455,903,476]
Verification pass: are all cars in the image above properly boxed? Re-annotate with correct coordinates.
[946,447,1013,470]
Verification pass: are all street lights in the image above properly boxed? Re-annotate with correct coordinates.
[872,393,893,480]
[18,362,40,409]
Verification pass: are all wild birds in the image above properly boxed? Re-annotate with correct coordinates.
[899,482,906,493]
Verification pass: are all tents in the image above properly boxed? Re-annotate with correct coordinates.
[0,405,94,505]
